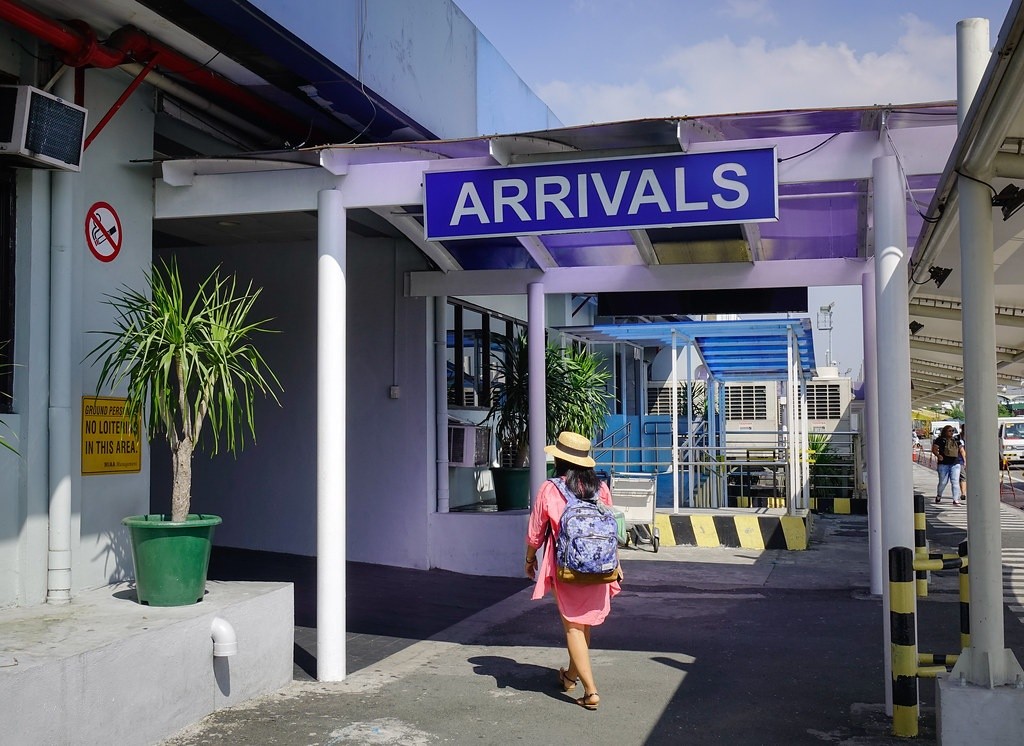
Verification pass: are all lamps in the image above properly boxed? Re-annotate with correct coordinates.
[909,258,953,289]
[909,320,924,335]
[953,166,1024,221]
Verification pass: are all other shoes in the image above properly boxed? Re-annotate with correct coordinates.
[953,501,961,507]
[935,497,942,504]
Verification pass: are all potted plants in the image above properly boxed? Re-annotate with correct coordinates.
[77,253,286,606]
[465,317,621,512]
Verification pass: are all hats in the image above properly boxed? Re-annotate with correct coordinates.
[543,431,597,468]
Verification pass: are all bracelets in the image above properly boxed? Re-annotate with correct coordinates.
[526,556,536,563]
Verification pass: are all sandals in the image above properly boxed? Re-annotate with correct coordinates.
[559,670,577,692]
[575,692,600,710]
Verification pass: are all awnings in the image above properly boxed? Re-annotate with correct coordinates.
[912,409,952,421]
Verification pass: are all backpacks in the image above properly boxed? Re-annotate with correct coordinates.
[548,477,620,576]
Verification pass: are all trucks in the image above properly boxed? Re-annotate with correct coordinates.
[929,421,961,451]
[998,418,1024,469]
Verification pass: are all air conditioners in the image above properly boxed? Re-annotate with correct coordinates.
[0,84,89,174]
[447,424,493,468]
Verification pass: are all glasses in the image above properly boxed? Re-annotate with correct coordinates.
[946,429,955,432]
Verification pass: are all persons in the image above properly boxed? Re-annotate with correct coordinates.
[526,432,623,710]
[932,425,967,506]
[955,424,967,502]
[912,429,917,447]
